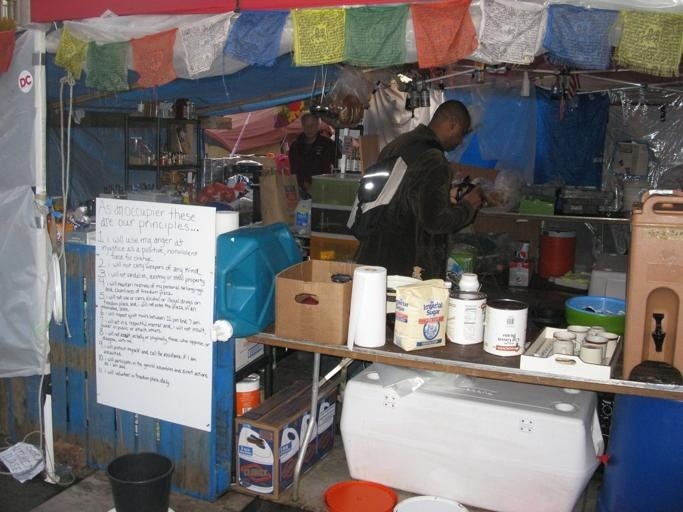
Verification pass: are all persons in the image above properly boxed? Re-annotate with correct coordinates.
[320,126,333,140]
[288,113,342,200]
[333,100,482,436]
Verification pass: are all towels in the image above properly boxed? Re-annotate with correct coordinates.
[107,452,174,512]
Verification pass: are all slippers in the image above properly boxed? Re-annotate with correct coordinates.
[344,154,410,247]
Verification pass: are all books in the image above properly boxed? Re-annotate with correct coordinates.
[233,258,365,500]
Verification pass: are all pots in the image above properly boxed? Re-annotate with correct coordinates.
[564,295,625,336]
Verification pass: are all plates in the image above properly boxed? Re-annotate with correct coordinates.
[123,116,201,203]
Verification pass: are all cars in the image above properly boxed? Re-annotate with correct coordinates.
[552,325,618,366]
[136,95,145,113]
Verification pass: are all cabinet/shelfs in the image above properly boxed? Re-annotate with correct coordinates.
[183,97,195,120]
[337,147,362,174]
[409,76,430,108]
[551,78,560,99]
[474,61,486,84]
[128,136,184,165]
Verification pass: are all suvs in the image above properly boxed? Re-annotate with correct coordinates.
[279,428,302,492]
[300,413,318,466]
[447,290,487,345]
[108,453,174,512]
[318,399,335,457]
[324,482,396,512]
[540,233,575,279]
[484,299,529,356]
[216,221,303,337]
[392,496,468,512]
[237,425,272,493]
[294,193,311,237]
[236,374,260,416]
